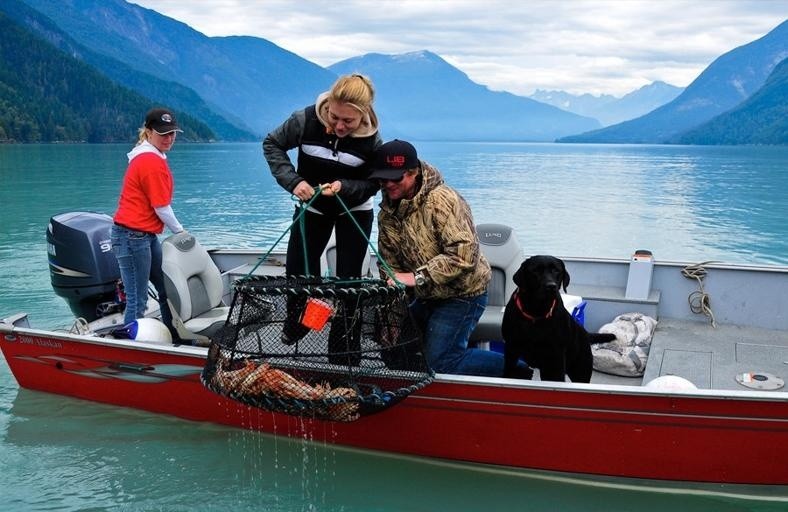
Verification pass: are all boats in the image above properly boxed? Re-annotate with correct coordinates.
[0,208,788,486]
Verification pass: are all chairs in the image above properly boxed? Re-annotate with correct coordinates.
[160,232,269,347]
[472,223,523,351]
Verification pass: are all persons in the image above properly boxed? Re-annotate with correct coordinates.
[368,138,531,378]
[261,72,384,367]
[110,109,194,345]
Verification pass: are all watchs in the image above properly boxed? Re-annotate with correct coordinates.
[413,270,425,286]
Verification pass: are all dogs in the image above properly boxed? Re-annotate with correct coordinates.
[499,253,617,382]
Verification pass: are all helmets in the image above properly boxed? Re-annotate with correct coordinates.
[112,317,172,343]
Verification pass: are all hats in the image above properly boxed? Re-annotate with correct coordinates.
[145,108,184,136]
[368,139,418,181]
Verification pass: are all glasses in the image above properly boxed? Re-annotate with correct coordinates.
[378,175,406,183]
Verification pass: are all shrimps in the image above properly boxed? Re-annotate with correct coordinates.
[209,354,363,424]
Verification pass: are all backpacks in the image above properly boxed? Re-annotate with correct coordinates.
[590,312,657,378]
